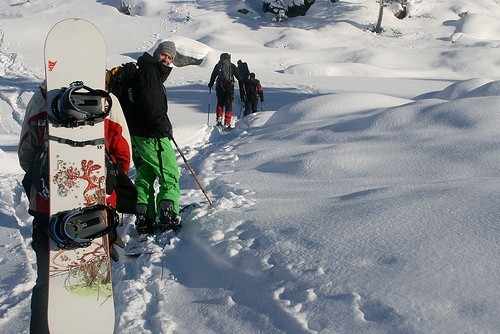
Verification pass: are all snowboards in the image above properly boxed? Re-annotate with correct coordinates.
[43,18,116,334]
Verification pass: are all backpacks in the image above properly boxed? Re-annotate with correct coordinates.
[106,61,144,134]
[217,60,232,83]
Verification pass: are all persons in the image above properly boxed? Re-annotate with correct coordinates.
[18,78,134,334]
[236,60,250,101]
[243,73,264,116]
[207,52,244,131]
[117,40,183,233]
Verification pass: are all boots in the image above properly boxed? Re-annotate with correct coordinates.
[158,199,182,233]
[217,117,222,126]
[224,125,231,130]
[133,203,158,236]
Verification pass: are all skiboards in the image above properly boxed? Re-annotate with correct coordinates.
[124,203,201,256]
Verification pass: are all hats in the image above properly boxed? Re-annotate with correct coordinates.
[153,41,177,62]
[220,53,231,59]
